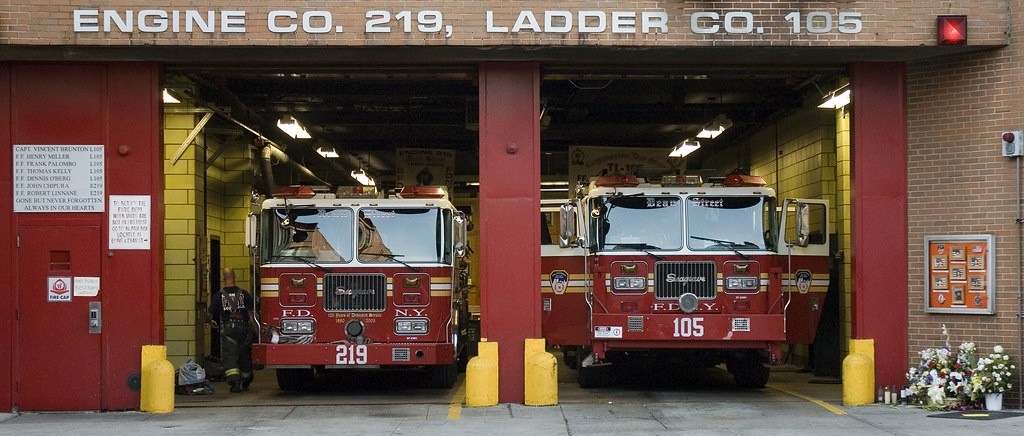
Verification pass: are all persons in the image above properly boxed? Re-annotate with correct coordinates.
[764,230,773,250]
[206,267,254,393]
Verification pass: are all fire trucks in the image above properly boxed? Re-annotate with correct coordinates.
[540,175,829,388]
[251,185,473,392]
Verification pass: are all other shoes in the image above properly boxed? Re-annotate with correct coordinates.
[230,381,239,392]
[240,381,246,391]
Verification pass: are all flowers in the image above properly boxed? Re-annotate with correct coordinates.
[905,324,976,408]
[970,345,1015,401]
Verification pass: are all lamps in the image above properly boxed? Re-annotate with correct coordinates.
[818,77,851,109]
[276,115,312,139]
[312,126,339,158]
[696,91,733,138]
[667,126,701,157]
[351,158,375,186]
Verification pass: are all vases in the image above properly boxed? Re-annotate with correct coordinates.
[927,398,959,408]
[985,393,1002,411]
